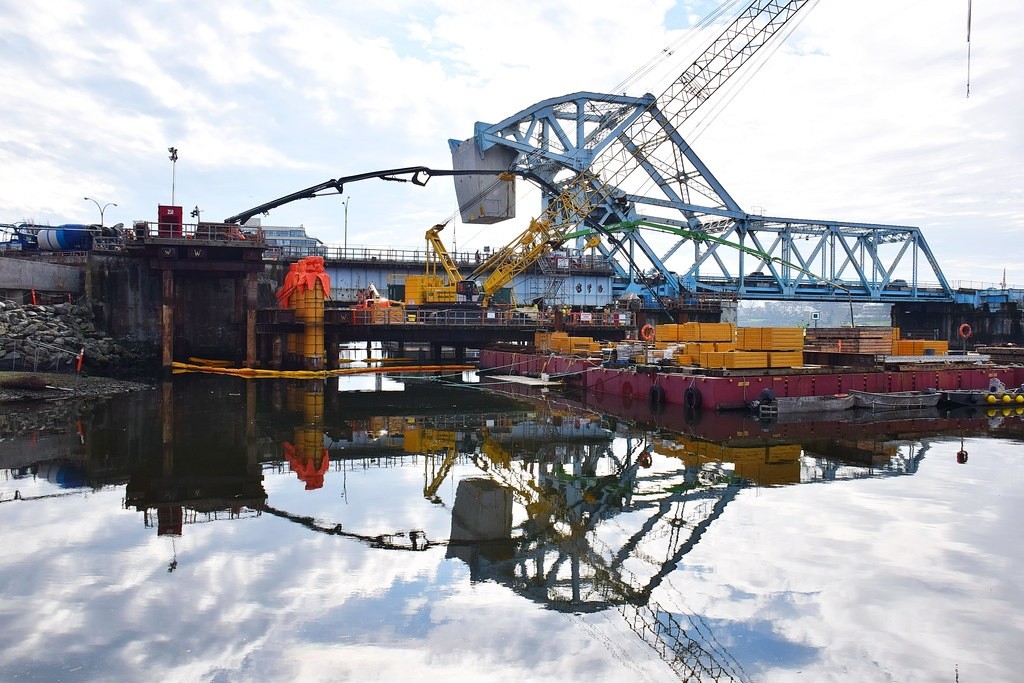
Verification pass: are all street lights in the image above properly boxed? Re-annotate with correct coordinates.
[84,197,119,226]
[191,206,201,223]
[342,196,350,256]
[166,146,179,207]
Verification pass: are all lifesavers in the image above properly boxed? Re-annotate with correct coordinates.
[684,387,701,407]
[641,452,654,470]
[650,386,665,401]
[641,324,656,339]
[649,401,664,415]
[959,322,972,337]
[683,408,703,426]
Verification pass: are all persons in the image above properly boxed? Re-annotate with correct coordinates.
[604,304,611,323]
[545,304,572,322]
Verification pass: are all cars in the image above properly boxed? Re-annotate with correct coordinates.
[893,279,909,287]
[749,271,765,277]
[652,271,679,278]
[817,279,845,288]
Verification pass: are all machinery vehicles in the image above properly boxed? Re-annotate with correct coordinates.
[551,461,752,498]
[553,218,857,327]
[401,415,765,682]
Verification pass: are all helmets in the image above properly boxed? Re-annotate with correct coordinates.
[547,306,551,308]
[564,306,567,308]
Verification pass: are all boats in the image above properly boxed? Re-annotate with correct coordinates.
[760,393,856,414]
[848,387,942,411]
[937,384,1024,407]
